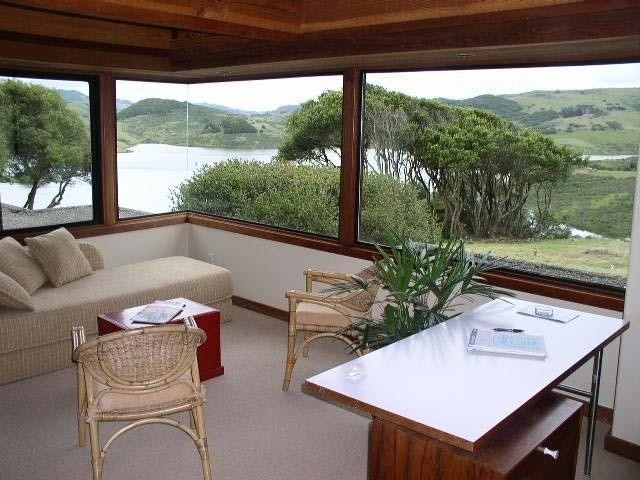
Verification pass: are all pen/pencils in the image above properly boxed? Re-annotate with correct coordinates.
[492,327,524,333]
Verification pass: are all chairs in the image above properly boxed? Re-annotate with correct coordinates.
[70,314,215,480]
[280,256,395,394]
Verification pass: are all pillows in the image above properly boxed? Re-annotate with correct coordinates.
[0,271,36,312]
[0,236,49,297]
[23,226,94,289]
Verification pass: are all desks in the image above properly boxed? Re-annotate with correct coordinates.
[300,296,632,480]
[95,296,226,384]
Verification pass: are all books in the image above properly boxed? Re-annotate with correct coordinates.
[130,299,184,325]
[468,329,548,362]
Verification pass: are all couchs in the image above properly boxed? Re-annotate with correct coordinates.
[0,244,236,389]
